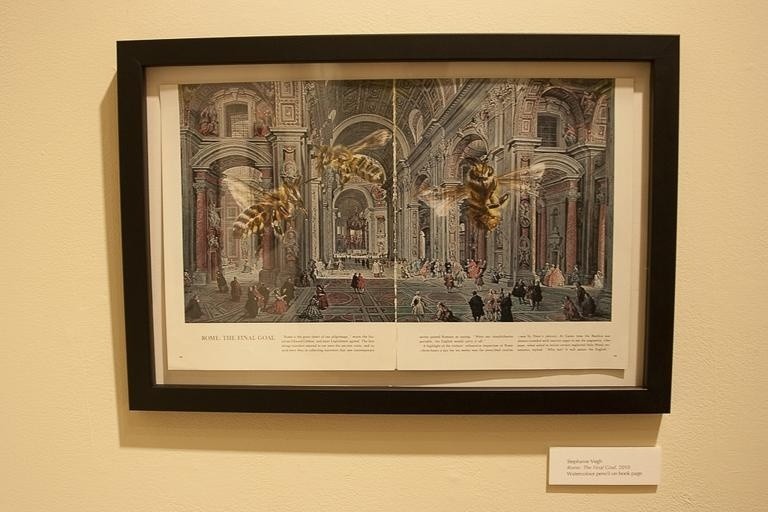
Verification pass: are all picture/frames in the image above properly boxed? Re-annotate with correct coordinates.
[117,34,680,414]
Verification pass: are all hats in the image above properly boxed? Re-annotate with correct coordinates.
[414,291,423,294]
